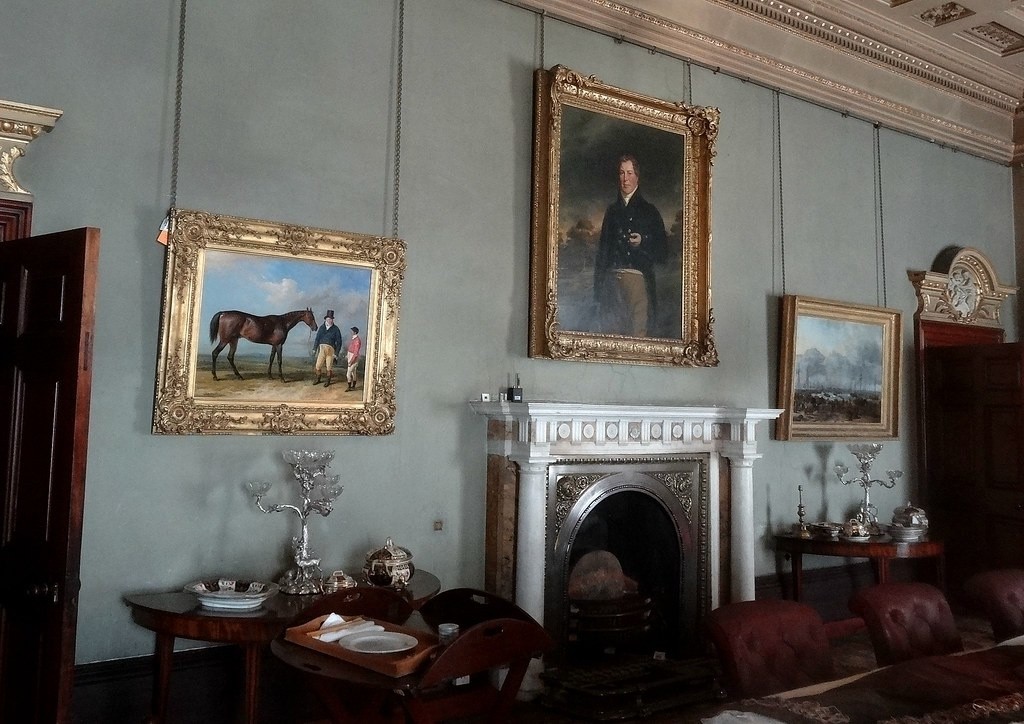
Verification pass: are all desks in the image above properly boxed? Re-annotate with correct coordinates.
[692,634,1024,724]
[122,568,453,724]
[772,524,944,604]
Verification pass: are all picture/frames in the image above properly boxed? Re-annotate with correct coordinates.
[775,294,904,443]
[528,64,721,370]
[151,208,408,438]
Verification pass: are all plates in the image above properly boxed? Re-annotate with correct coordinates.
[837,533,871,541]
[339,631,419,653]
[185,578,283,609]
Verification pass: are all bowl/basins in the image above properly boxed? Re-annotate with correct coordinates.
[890,528,923,542]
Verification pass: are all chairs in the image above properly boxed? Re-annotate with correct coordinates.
[967,569,1024,641]
[700,598,867,699]
[270,587,552,724]
[848,582,965,668]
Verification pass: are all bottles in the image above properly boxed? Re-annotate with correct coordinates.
[438,623,459,654]
